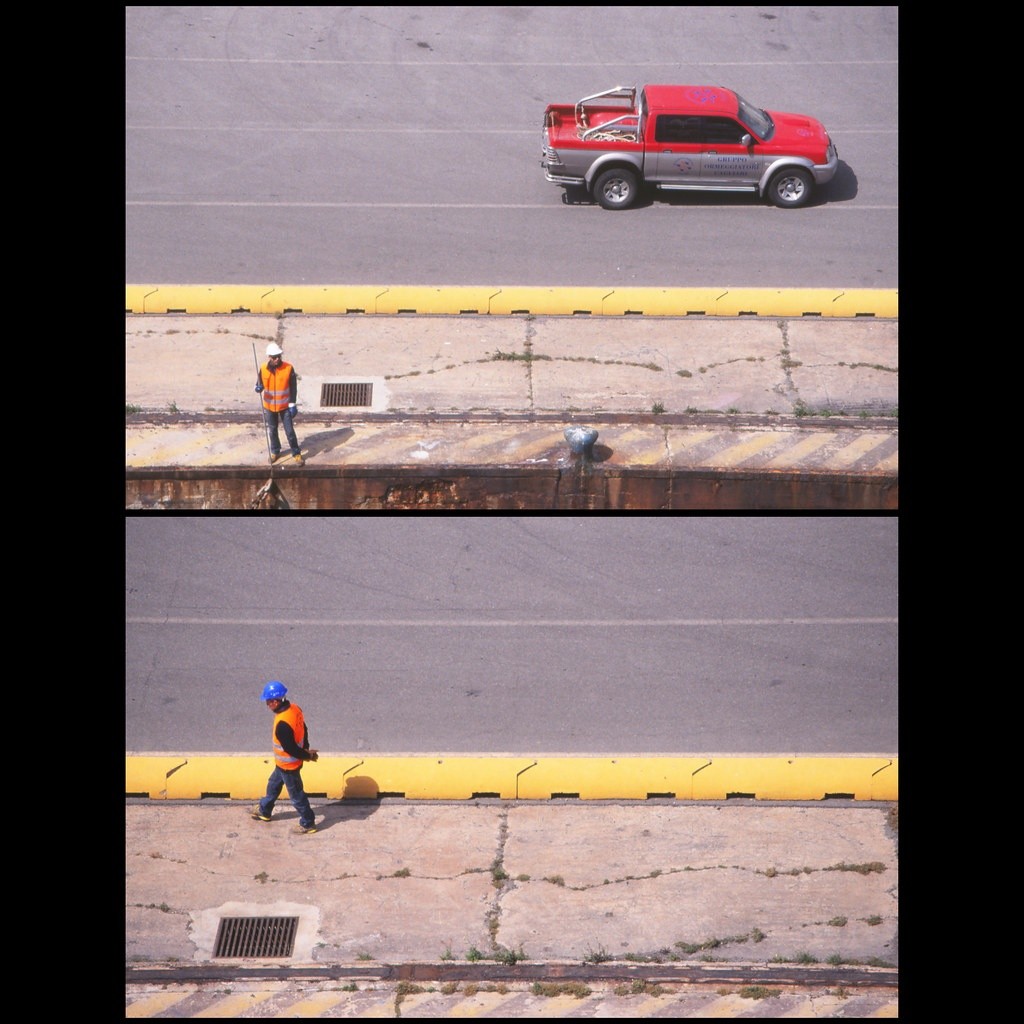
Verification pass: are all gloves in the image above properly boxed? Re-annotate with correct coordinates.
[289,405,298,417]
[304,749,319,762]
[256,386,263,394]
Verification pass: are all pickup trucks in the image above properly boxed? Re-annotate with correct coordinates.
[540,84,842,209]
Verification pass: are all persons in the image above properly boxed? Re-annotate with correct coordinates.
[246,681,318,834]
[255,343,305,467]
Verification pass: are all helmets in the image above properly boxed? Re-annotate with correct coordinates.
[259,680,287,700]
[266,343,283,356]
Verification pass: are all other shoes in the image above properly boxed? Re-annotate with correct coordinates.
[295,454,304,466]
[269,454,277,463]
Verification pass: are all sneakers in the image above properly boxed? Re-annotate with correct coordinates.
[289,826,317,833]
[247,806,271,821]
